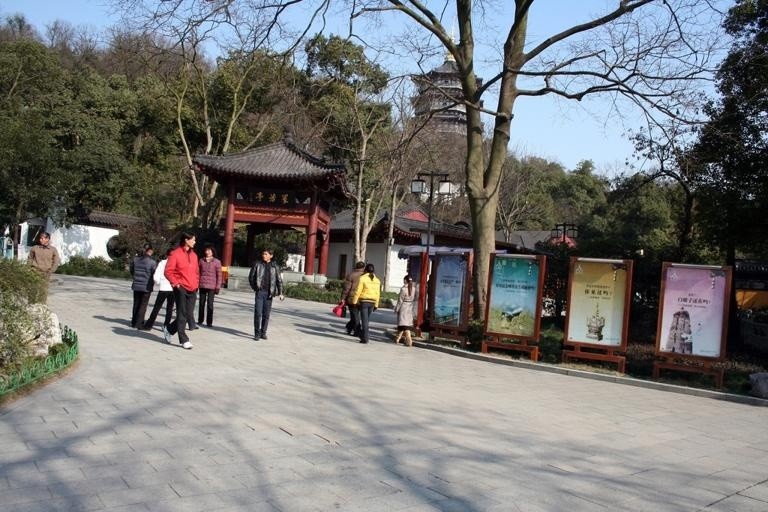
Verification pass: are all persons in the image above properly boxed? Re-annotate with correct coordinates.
[341,262,365,337]
[249,248,285,341]
[197,245,222,327]
[352,264,381,343]
[394,275,416,347]
[162,231,200,349]
[130,244,158,330]
[188,317,199,330]
[27,232,59,304]
[144,248,175,331]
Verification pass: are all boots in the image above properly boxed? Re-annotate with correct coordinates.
[403,330,411,346]
[392,330,404,343]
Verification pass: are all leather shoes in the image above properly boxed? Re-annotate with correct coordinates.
[261,334,267,339]
[207,323,211,327]
[198,321,202,325]
[253,337,259,341]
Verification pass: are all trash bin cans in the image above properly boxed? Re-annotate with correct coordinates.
[222,266,229,288]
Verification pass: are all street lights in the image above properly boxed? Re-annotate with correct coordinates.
[410,168,453,339]
[548,221,581,320]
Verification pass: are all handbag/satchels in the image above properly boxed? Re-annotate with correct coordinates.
[341,307,345,317]
[332,303,344,316]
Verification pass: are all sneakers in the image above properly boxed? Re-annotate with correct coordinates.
[161,325,171,343]
[182,342,193,349]
